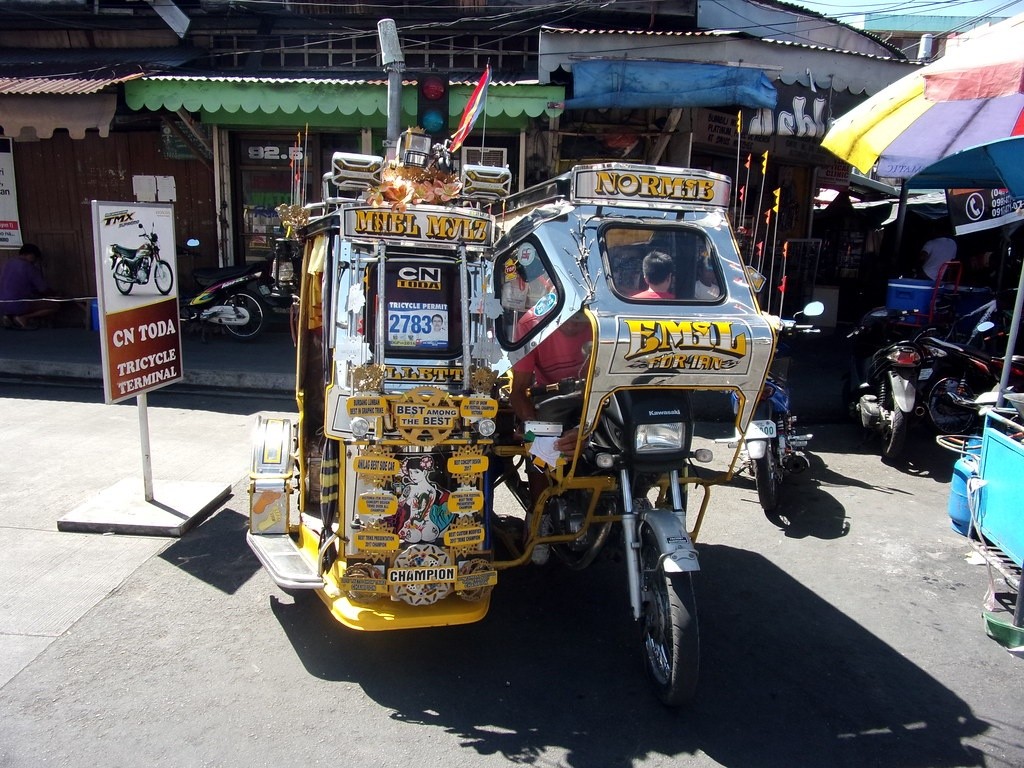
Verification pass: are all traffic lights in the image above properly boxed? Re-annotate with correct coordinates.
[415,71,449,149]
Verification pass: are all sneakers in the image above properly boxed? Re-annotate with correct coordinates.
[523,504,554,566]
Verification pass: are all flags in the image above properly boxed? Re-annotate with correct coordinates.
[449,67,492,153]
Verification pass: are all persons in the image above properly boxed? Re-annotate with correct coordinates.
[509,285,654,565]
[915,224,957,282]
[432,315,443,331]
[0,244,59,329]
[631,251,677,299]
[695,256,720,300]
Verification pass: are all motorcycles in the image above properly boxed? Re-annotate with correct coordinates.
[185,234,296,342]
[110,222,174,297]
[841,309,1024,460]
[722,300,825,511]
[245,132,775,711]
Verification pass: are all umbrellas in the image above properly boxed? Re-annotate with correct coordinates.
[924,26,1024,103]
[818,66,1024,407]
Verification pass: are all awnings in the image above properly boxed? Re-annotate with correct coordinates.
[0,45,206,140]
[124,71,565,130]
